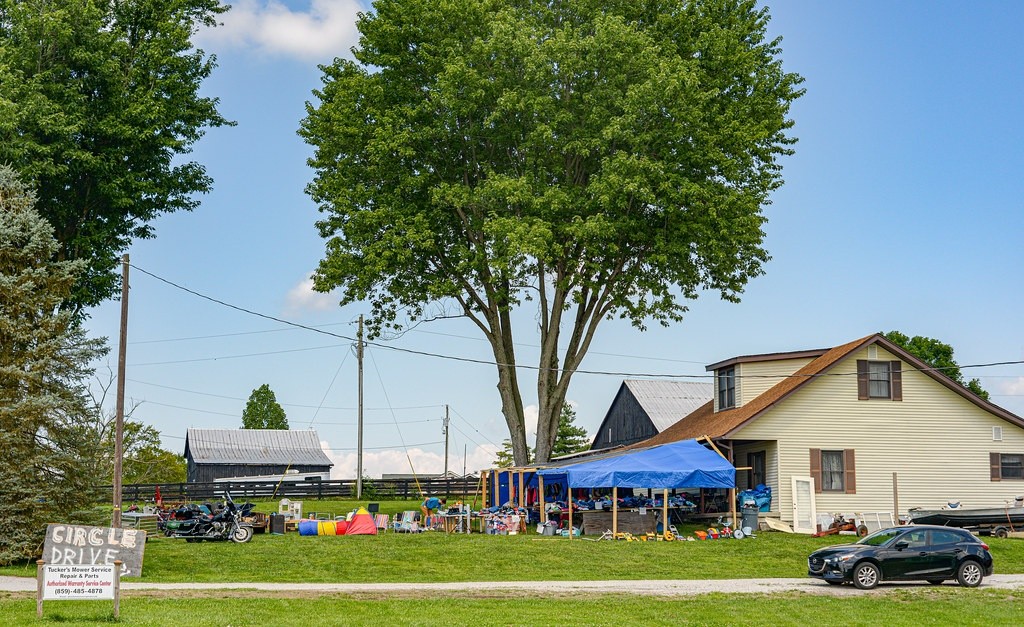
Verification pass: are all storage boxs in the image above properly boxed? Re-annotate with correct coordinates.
[537,523,558,535]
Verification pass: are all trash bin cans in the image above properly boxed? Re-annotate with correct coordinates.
[270,514,285,534]
[546,524,558,537]
[742,507,759,532]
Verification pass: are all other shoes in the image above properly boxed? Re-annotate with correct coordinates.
[430,528,435,531]
[424,528,429,531]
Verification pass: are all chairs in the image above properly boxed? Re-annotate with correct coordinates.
[705,492,725,513]
[373,514,389,535]
[394,510,415,534]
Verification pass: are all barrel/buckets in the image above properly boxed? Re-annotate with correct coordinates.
[270,514,284,534]
[741,506,758,531]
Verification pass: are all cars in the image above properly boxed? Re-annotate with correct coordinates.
[807,523,994,590]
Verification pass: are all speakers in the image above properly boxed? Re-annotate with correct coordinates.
[270,514,283,533]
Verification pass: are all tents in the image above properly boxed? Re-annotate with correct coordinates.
[480,435,736,540]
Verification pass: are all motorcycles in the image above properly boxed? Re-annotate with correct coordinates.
[157,491,256,544]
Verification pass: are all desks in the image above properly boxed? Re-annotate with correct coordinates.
[498,515,526,534]
[283,520,298,534]
[562,507,692,536]
[439,515,485,534]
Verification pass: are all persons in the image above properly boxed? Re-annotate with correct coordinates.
[420,498,446,531]
